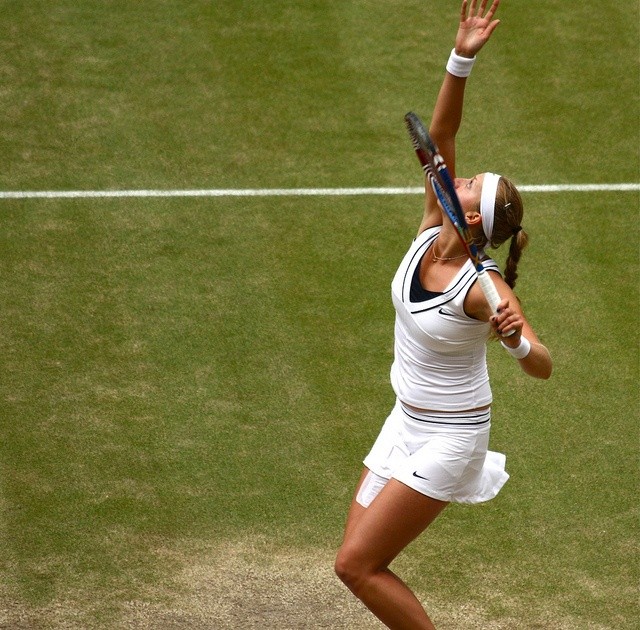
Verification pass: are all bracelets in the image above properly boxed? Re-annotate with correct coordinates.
[446,49,478,79]
[500,336,531,360]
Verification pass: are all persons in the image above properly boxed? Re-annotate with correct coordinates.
[335,0,552,630]
